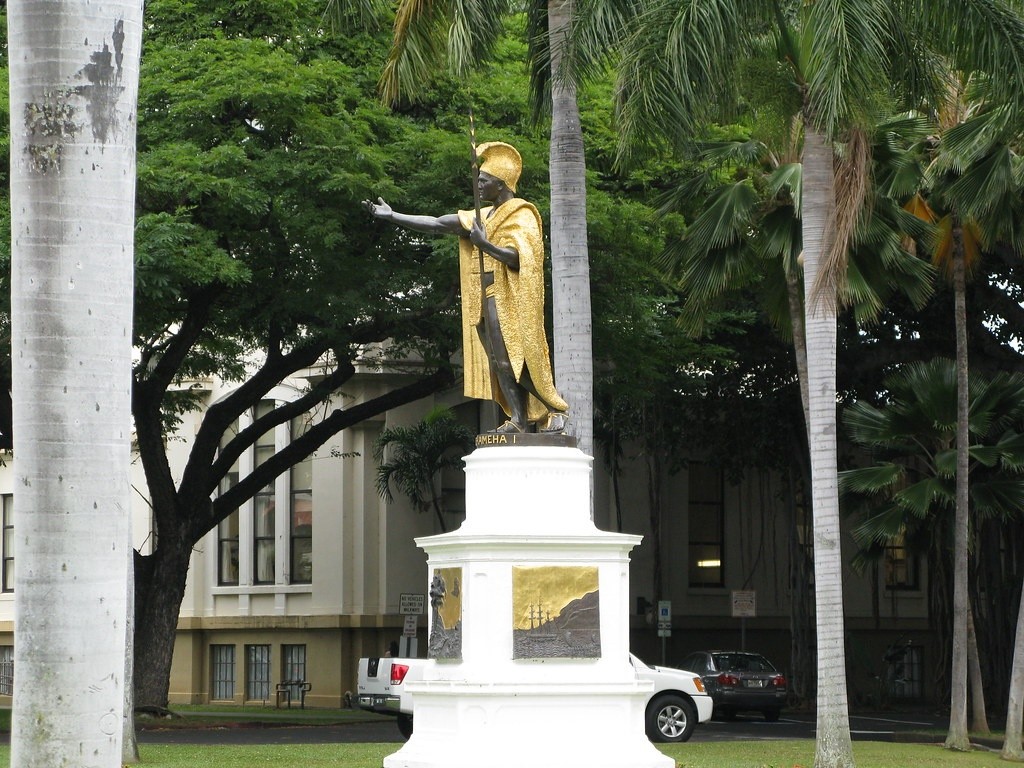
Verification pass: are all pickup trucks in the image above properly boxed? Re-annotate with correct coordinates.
[357,649,714,744]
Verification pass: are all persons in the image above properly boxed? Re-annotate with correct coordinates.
[385,641,398,657]
[360,141,570,433]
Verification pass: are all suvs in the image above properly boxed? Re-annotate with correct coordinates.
[668,648,788,722]
